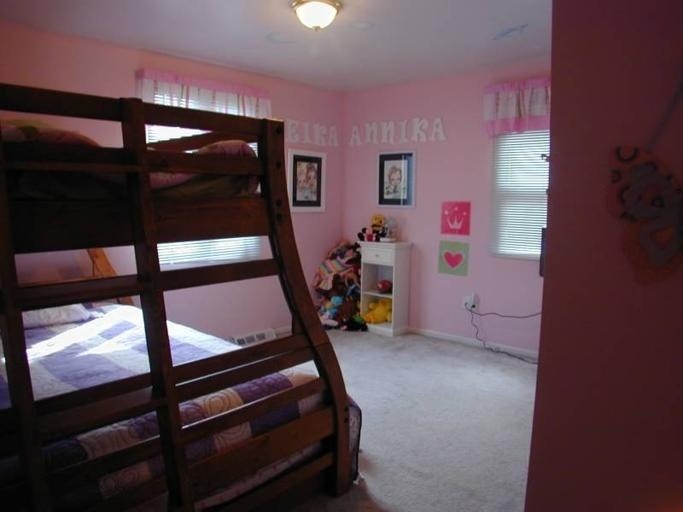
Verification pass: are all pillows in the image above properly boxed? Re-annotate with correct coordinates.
[22,300,91,329]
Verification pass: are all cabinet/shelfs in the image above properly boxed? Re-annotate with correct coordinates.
[355,238,414,338]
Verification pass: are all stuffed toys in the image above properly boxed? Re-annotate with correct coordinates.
[356,212,401,243]
[360,297,392,326]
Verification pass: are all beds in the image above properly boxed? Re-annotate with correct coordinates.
[0,80,363,511]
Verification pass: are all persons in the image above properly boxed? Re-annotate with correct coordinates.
[384,161,407,199]
[296,161,318,202]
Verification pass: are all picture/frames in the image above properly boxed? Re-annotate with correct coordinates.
[377,150,415,206]
[286,148,328,213]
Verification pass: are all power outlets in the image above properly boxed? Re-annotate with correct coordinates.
[463,296,478,311]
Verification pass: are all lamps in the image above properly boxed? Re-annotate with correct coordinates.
[290,0,343,31]
